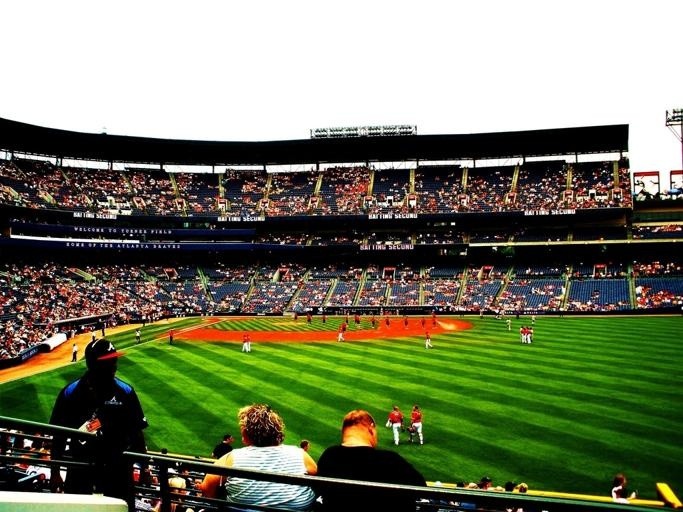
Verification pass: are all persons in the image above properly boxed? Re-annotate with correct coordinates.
[1,156,683,511]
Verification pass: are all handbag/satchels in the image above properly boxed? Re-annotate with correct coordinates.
[408,427,416,432]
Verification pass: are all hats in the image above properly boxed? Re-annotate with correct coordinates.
[482,477,490,482]
[85,338,123,360]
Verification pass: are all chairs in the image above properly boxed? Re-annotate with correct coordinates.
[0,149,682,238]
[0,259,130,371]
[131,263,683,318]
